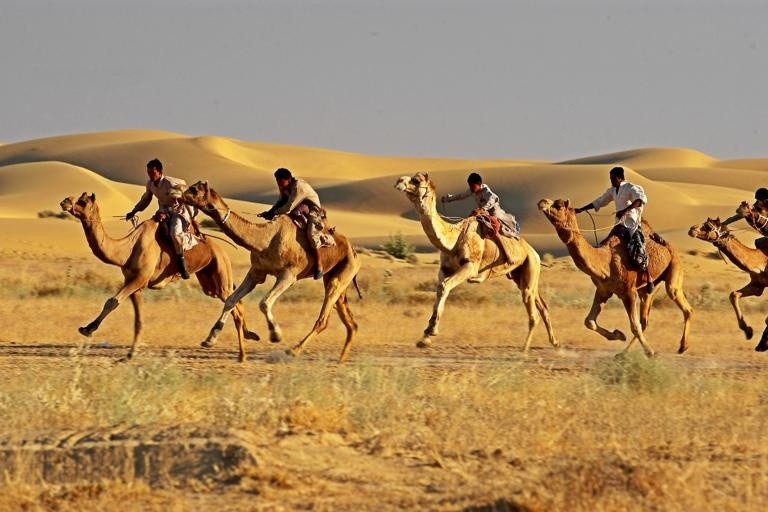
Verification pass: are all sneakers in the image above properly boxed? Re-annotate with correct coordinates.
[647,282,654,294]
[314,271,323,280]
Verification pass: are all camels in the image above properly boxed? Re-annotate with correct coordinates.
[59,191,260,365]
[688,200,768,353]
[177,180,362,365]
[537,198,695,359]
[393,171,559,356]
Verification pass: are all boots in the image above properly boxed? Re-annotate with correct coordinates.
[177,254,190,279]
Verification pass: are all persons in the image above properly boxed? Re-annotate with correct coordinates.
[722,188,768,255]
[257,168,324,280]
[125,158,200,279]
[574,167,654,294]
[442,172,516,265]
